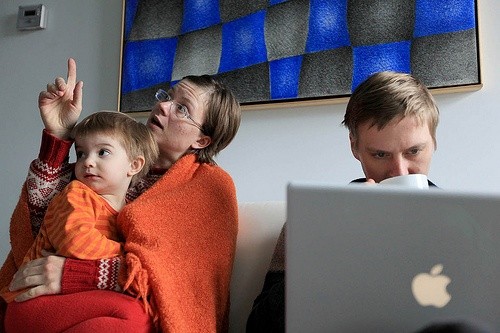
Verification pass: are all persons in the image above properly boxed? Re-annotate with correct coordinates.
[4,110,160,333]
[246,70,440,333]
[0,57,241,333]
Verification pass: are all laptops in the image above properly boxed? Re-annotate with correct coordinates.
[286,182,500,333]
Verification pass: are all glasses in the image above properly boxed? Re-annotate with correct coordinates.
[154,88,210,135]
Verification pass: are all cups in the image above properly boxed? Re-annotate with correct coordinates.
[374,174,429,189]
